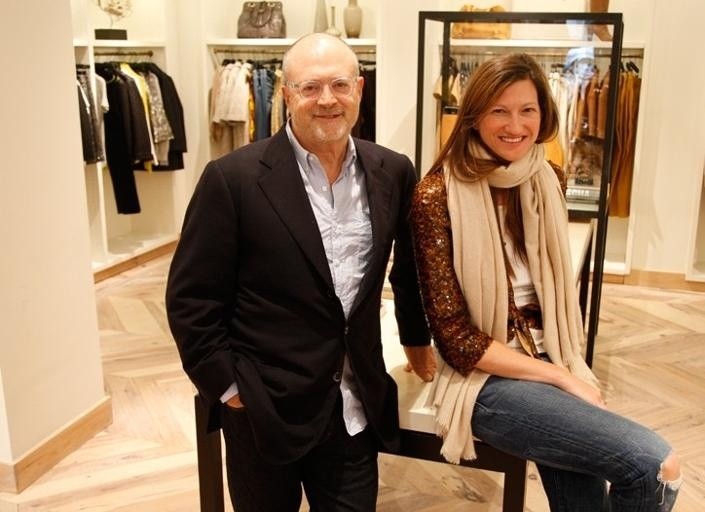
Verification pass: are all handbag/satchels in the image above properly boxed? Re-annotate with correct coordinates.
[452,5,512,39]
[238,2,285,38]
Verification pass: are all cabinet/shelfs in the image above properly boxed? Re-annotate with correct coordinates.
[73,40,176,275]
[434,38,651,276]
[204,39,383,160]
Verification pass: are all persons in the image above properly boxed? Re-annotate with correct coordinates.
[164,31,437,512]
[407,51,682,512]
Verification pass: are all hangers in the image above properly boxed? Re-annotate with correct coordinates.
[358,51,376,70]
[96,51,151,64]
[222,50,282,65]
[440,53,640,76]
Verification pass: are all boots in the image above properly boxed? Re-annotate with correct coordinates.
[588,0,613,41]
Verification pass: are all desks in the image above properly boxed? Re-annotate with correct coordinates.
[194,218,595,512]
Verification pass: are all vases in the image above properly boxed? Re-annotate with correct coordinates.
[343,0,362,39]
[314,1,327,34]
[325,6,342,37]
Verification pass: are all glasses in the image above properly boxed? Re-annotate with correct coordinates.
[288,78,356,100]
